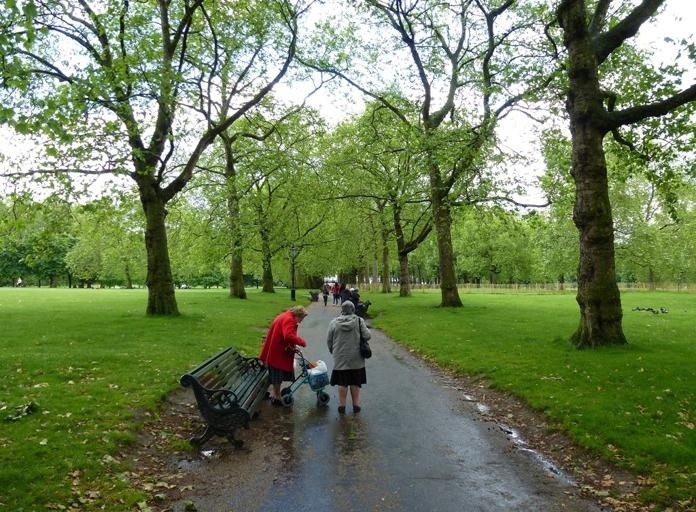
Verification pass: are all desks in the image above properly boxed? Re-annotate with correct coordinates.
[289,246,297,300]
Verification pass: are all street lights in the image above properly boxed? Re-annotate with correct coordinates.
[358,318,372,359]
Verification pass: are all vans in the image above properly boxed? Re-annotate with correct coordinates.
[338,406,345,414]
[353,405,360,412]
[271,399,282,408]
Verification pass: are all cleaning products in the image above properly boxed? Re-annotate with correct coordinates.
[281,344,331,407]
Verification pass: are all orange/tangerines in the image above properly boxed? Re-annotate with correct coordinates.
[355,298,372,317]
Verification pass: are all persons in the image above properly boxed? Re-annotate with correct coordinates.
[260,305,307,407]
[323,281,360,306]
[327,300,372,413]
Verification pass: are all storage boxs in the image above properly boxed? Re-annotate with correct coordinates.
[179,345,275,450]
